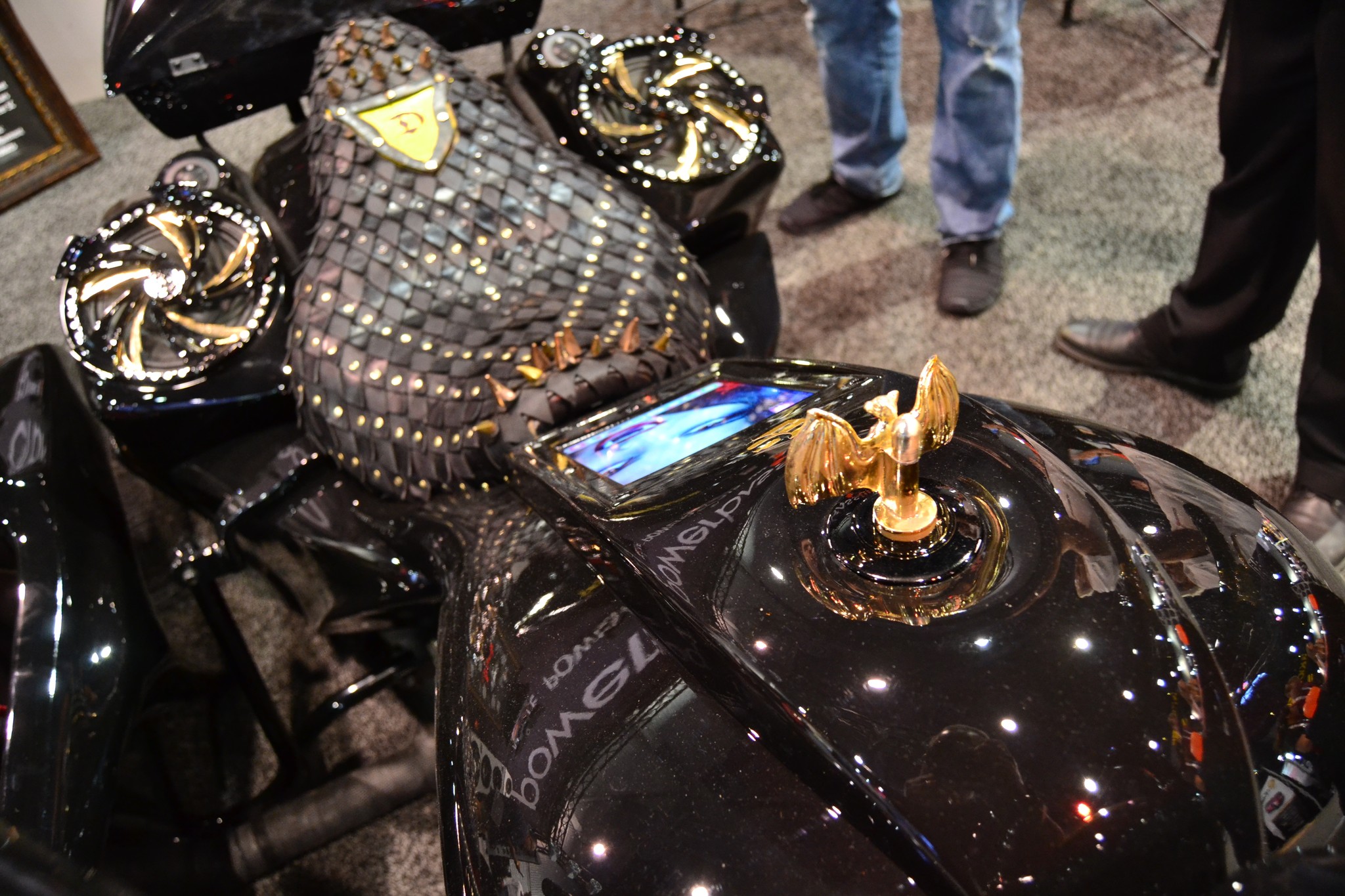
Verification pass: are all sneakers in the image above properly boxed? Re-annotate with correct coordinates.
[937,228,1008,317]
[781,181,902,235]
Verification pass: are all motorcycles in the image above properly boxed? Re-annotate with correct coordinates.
[0,3,1345,896]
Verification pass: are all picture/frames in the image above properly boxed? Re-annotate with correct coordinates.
[1,0,105,217]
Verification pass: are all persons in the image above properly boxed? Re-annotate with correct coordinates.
[1055,0,1345,569]
[778,1,1025,314]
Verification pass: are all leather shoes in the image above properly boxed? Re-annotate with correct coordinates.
[1058,317,1248,398]
[1281,487,1345,574]
